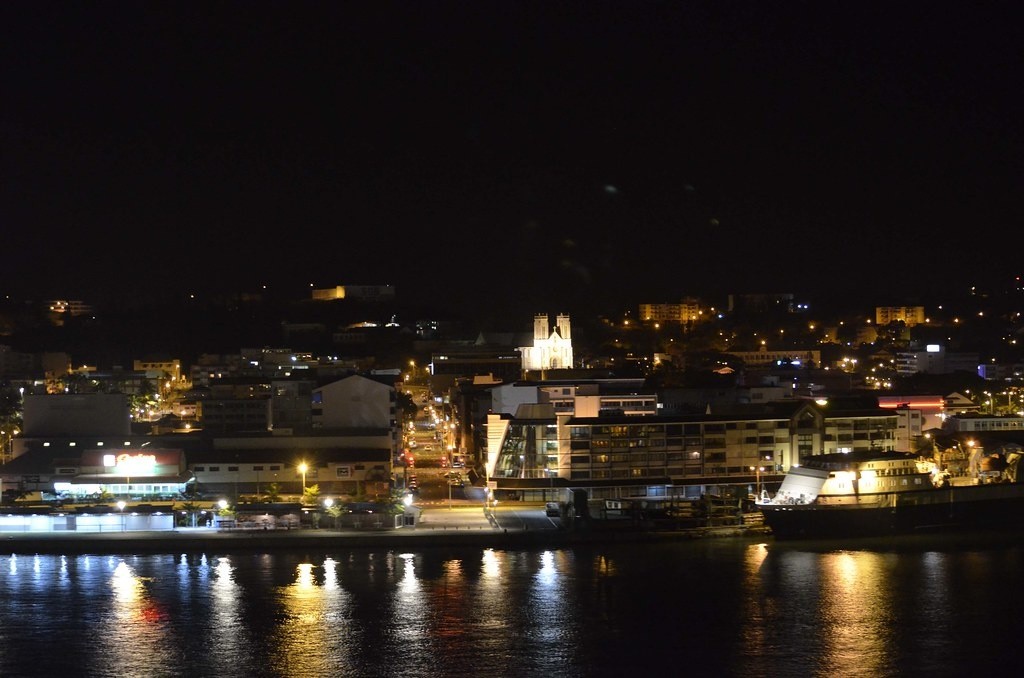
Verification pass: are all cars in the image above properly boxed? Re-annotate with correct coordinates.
[407,421,417,490]
[428,425,464,487]
[545,502,560,518]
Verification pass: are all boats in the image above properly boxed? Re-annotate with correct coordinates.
[754,448,1024,543]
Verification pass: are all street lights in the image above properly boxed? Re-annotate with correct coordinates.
[925,434,935,455]
[176,470,197,505]
[545,469,560,501]
[297,461,307,501]
[750,465,764,502]
